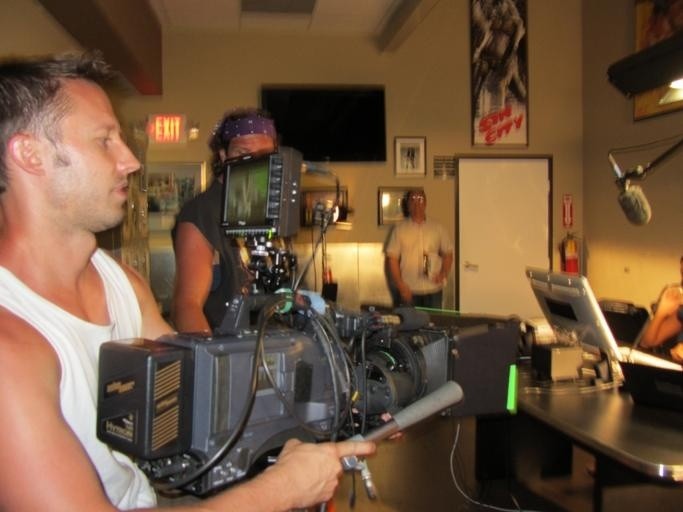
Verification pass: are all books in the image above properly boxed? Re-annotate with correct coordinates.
[422,252,448,287]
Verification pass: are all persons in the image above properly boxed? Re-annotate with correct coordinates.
[641,254,683,364]
[1,48,377,512]
[404,144,417,169]
[383,188,453,309]
[169,109,308,333]
[472,1,528,119]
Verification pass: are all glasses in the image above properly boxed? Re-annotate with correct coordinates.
[408,197,425,203]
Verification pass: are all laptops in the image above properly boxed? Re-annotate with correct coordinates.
[525,265,683,381]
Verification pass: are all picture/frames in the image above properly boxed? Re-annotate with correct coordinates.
[299,185,349,224]
[378,185,425,226]
[393,136,427,178]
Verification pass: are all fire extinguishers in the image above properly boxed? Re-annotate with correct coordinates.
[558,231,581,274]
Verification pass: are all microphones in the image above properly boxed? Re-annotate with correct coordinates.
[606,153,652,225]
[337,379,465,463]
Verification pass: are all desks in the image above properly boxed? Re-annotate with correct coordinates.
[491,327,682,512]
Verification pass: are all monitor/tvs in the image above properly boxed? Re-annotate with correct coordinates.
[261,85,386,161]
